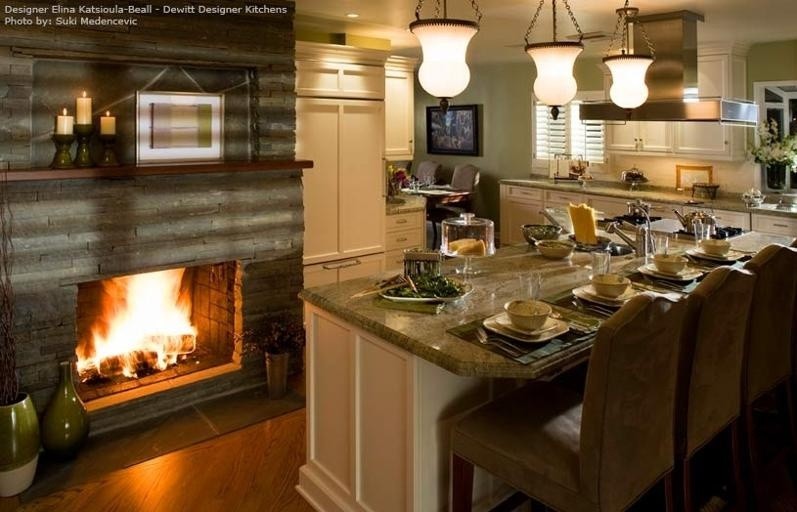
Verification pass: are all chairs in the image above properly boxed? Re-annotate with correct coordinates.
[406,158,443,189]
[744,242,797,500]
[676,264,758,512]
[424,161,481,251]
[443,289,695,512]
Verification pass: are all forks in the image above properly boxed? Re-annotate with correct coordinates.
[573,294,614,315]
[472,329,519,358]
[477,324,529,354]
[571,300,615,316]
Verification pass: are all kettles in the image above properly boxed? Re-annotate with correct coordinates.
[672,200,718,237]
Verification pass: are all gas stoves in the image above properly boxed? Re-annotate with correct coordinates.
[595,215,744,243]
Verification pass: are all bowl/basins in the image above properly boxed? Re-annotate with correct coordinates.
[591,273,631,298]
[533,239,577,259]
[653,252,689,274]
[504,299,553,331]
[700,239,732,255]
[520,223,563,246]
[569,234,611,252]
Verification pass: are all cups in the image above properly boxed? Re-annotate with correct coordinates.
[518,272,543,301]
[650,235,670,256]
[591,252,611,277]
[693,223,711,249]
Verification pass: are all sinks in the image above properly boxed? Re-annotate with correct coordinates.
[603,241,632,257]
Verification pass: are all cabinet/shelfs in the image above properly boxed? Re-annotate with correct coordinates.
[294,38,393,267]
[381,55,420,162]
[598,42,751,165]
[495,175,797,249]
[385,195,429,270]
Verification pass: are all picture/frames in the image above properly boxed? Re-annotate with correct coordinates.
[135,90,224,168]
[424,104,486,158]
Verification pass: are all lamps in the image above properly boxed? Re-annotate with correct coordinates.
[408,0,483,117]
[522,0,585,121]
[599,0,658,119]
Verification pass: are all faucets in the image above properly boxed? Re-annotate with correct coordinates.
[605,202,656,257]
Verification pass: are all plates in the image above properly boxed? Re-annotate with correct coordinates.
[692,247,738,258]
[582,285,637,302]
[684,249,747,262]
[482,311,571,344]
[495,314,562,337]
[635,264,705,282]
[374,282,476,303]
[570,284,640,307]
[642,263,695,278]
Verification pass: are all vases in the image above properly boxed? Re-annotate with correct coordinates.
[262,350,290,402]
[41,359,91,456]
[0,393,42,499]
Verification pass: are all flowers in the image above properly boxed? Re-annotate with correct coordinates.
[746,114,793,178]
[231,311,306,359]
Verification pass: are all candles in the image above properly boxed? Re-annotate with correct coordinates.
[56,106,74,137]
[100,110,116,135]
[76,90,93,127]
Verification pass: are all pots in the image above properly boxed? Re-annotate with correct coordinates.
[627,197,661,219]
